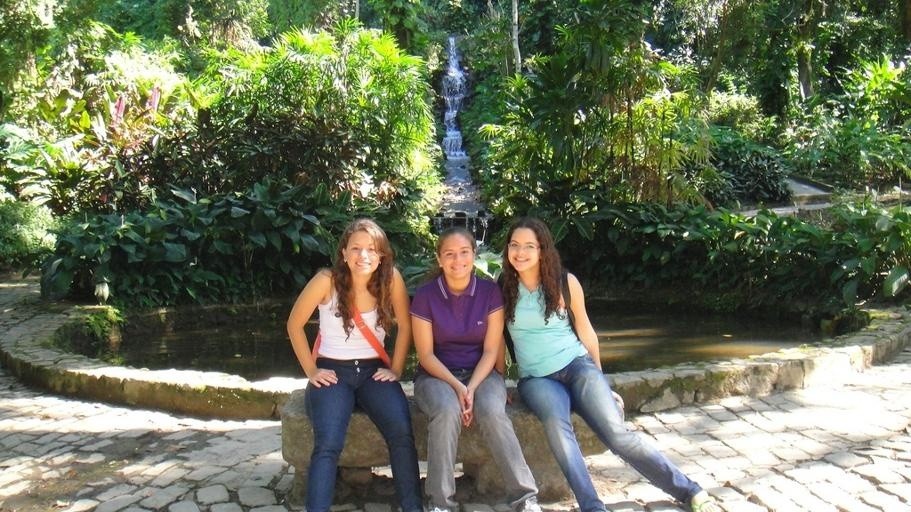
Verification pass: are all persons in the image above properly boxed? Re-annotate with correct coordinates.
[287,217,425,511]
[494,215,723,512]
[409,227,545,512]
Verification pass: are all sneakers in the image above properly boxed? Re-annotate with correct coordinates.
[429,500,544,512]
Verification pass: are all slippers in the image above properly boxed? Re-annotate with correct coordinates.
[690,489,725,512]
